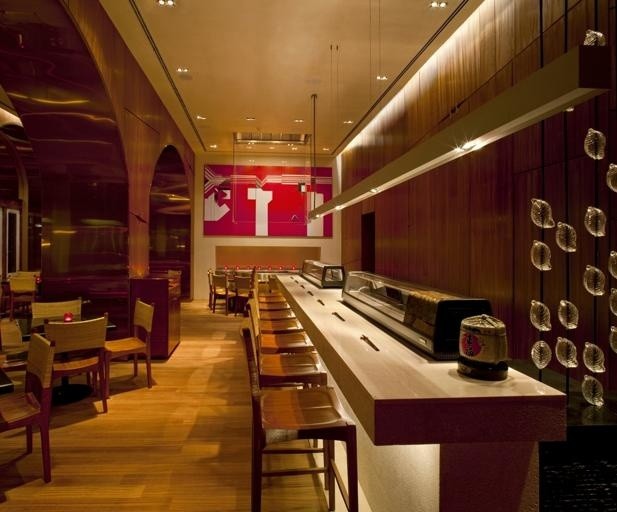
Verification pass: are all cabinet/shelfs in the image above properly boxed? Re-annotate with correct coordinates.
[131,271,181,359]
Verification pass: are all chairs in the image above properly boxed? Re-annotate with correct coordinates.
[0,271,156,500]
[208,267,250,317]
[242,266,357,512]
